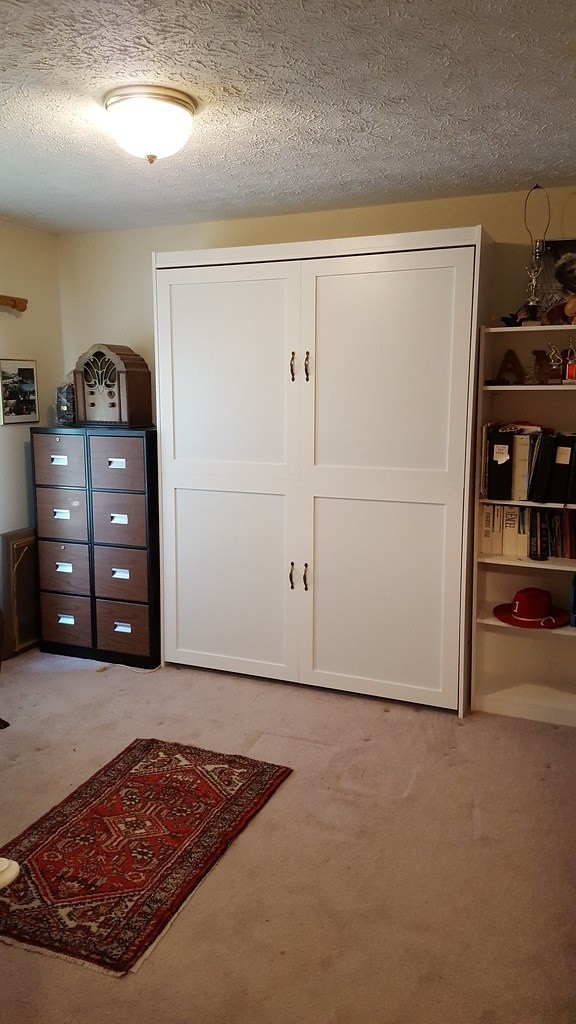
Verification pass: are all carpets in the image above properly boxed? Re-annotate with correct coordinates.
[0,738,293,978]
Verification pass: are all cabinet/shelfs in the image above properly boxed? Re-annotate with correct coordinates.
[471,323,576,730]
[30,425,158,670]
[158,226,490,717]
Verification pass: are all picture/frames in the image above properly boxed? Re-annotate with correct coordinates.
[0,359,40,425]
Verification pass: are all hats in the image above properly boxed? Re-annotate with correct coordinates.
[493,587,570,628]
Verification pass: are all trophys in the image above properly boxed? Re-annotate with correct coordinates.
[566,335,575,380]
[526,267,543,318]
[546,340,562,384]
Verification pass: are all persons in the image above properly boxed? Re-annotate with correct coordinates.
[554,253,576,296]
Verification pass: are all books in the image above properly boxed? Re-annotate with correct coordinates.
[478,420,575,560]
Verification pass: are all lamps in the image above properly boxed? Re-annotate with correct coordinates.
[104,84,197,164]
